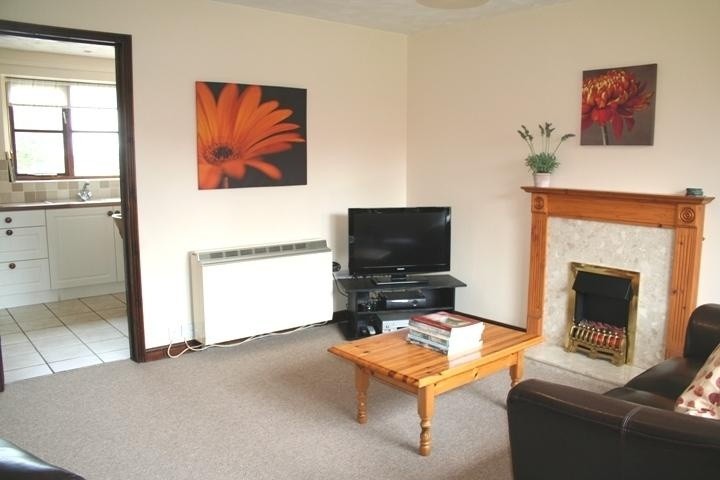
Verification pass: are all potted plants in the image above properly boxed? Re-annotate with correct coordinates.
[517,121,576,188]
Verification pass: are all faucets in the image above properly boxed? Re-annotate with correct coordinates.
[78,182,93,200]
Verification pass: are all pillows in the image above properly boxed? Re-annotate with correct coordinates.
[675,341,719,420]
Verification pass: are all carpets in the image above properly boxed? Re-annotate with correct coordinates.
[1,320,624,480]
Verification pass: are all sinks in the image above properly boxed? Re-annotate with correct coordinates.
[112,213,123,238]
[74,198,104,204]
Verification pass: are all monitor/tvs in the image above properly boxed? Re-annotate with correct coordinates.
[347,206,451,286]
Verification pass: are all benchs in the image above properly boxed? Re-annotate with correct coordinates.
[508,300,719,480]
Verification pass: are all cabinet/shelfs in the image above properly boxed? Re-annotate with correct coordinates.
[44,205,128,302]
[0,210,53,311]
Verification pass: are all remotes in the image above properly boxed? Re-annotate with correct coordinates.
[360,326,367,335]
[366,325,377,335]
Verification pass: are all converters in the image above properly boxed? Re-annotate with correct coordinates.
[333,262,341,272]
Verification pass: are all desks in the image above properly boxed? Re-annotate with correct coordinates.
[333,274,468,339]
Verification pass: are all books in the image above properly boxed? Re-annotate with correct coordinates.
[407,311,486,357]
[450,353,482,368]
